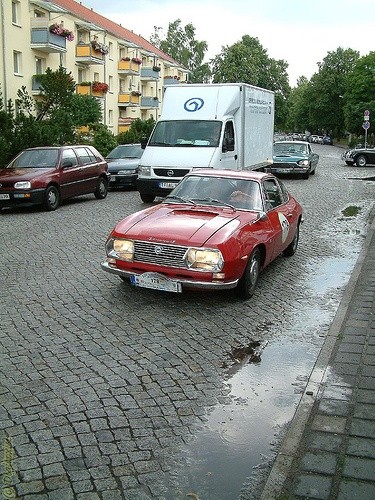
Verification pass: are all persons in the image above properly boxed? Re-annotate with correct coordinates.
[230,182,255,203]
[206,125,226,145]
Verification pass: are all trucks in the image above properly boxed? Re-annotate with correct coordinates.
[136,82,275,203]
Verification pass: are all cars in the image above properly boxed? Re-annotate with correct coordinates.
[273,131,334,145]
[0,144,111,212]
[342,146,375,167]
[264,140,320,180]
[104,143,145,190]
[99,167,304,302]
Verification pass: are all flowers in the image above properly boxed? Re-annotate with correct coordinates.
[153,66,161,72]
[92,40,110,54]
[131,58,143,64]
[93,81,109,93]
[49,23,75,41]
